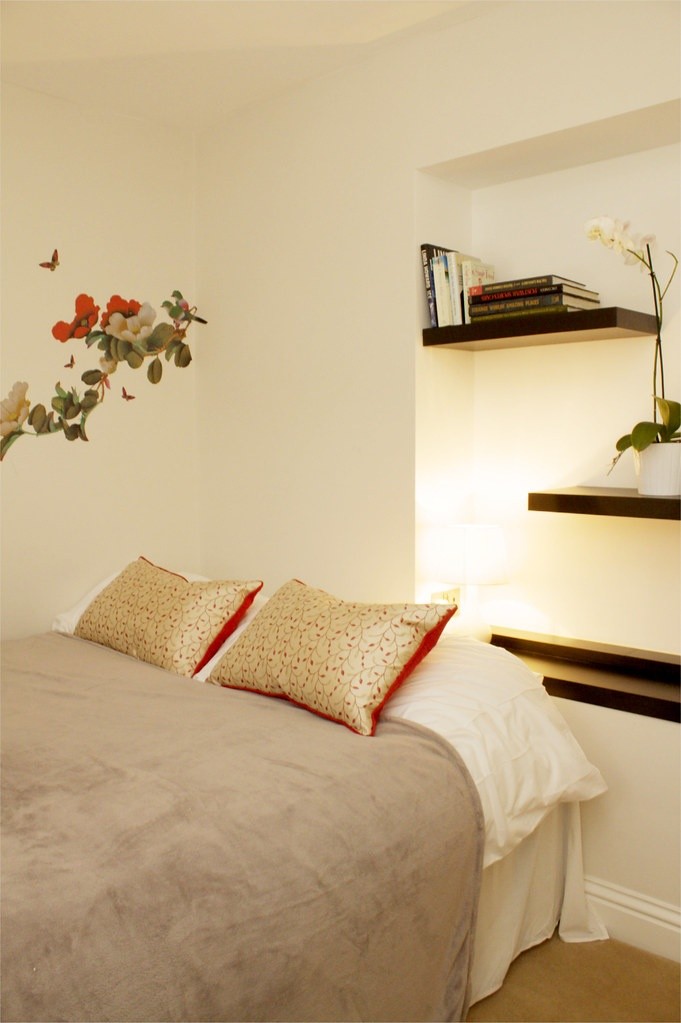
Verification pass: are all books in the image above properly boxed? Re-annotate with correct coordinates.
[420,242,496,329]
[466,274,602,324]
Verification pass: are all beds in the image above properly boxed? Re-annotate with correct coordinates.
[1,622,613,1022]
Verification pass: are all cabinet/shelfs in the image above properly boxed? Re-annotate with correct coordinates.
[422,305,681,523]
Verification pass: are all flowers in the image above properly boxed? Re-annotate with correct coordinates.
[585,213,681,476]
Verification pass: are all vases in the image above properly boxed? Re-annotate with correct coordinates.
[631,442,681,496]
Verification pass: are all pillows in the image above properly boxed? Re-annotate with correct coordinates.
[204,578,458,734]
[74,555,264,679]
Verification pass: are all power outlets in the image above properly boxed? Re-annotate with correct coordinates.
[428,585,462,618]
